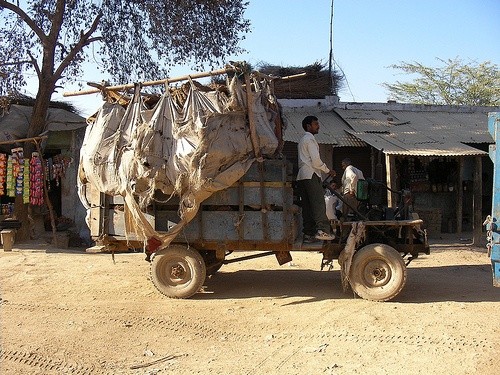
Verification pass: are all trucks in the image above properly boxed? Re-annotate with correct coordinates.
[86,159,431,302]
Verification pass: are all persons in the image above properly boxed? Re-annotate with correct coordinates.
[339,156,366,221]
[295,116,338,243]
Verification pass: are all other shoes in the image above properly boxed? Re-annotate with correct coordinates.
[303,233,318,242]
[315,232,336,240]
